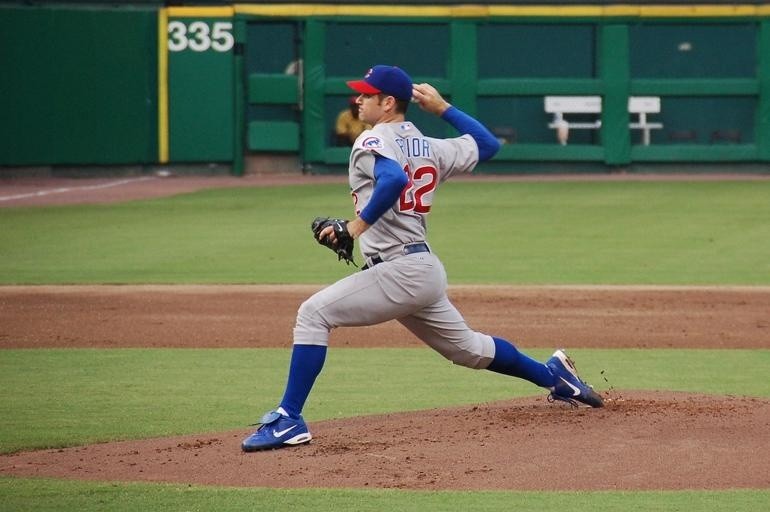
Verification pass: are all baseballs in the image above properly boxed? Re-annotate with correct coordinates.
[411,96,421,103]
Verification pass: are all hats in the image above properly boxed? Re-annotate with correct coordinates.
[346,64,414,101]
[349,95,358,105]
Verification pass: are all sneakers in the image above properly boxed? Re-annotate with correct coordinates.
[241,411,313,451]
[545,349,605,408]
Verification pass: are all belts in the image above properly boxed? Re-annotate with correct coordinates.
[361,243,429,270]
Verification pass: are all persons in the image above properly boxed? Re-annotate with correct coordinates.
[333,96,373,149]
[238,63,605,452]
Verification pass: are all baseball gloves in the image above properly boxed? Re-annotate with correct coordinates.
[312,217,357,268]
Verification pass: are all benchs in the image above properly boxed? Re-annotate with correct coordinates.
[542,93,664,146]
